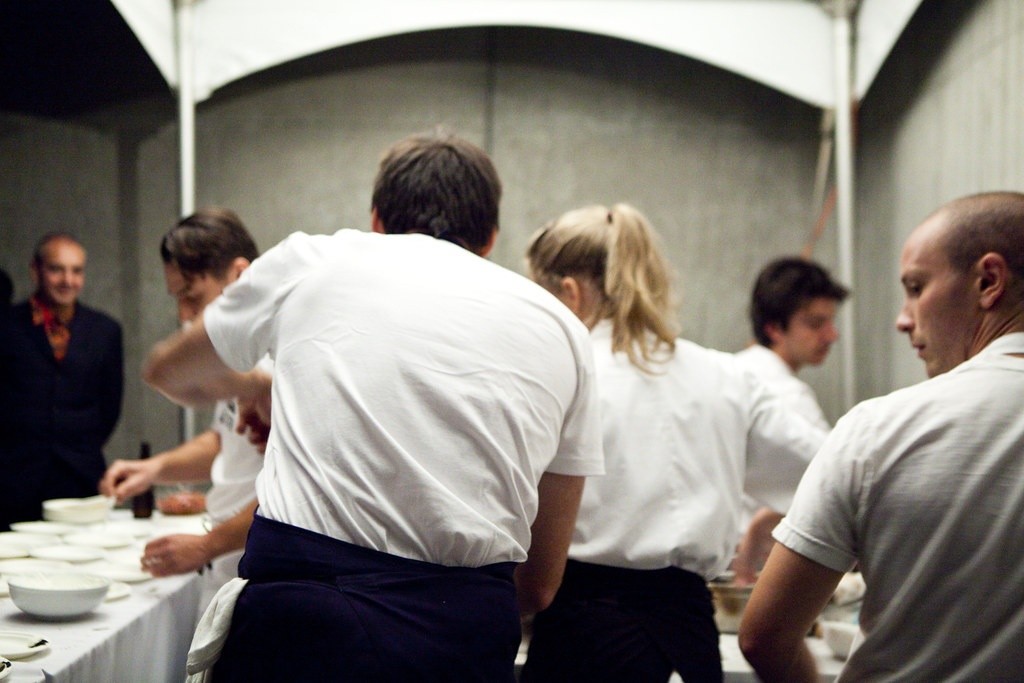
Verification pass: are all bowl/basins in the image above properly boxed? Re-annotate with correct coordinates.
[6,571,111,621]
[708,572,758,634]
[154,482,211,515]
[43,498,110,523]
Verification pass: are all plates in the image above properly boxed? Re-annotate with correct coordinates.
[0,510,213,680]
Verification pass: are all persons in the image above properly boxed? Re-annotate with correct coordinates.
[733,256,853,431]
[738,191,1024,682]
[141,129,606,683]
[524,204,829,683]
[0,230,126,532]
[99,205,273,683]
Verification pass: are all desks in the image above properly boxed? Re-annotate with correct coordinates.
[512,632,846,683]
[0,508,211,683]
